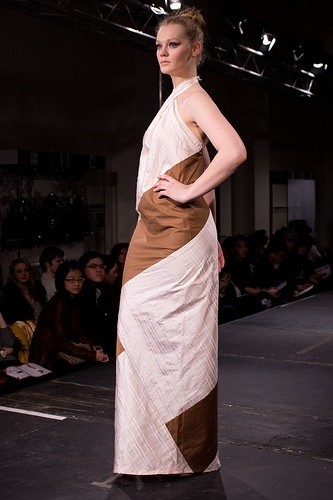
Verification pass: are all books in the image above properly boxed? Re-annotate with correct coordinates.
[4,363,52,380]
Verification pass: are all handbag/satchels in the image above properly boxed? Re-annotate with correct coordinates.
[59,341,91,365]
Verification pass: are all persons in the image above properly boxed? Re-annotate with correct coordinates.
[27,261,111,383]
[111,242,130,275]
[74,252,118,353]
[39,246,66,302]
[2,258,48,323]
[215,219,333,326]
[112,9,247,477]
[0,313,43,398]
[101,255,120,289]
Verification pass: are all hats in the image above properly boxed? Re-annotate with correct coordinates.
[279,227,294,241]
[225,234,249,248]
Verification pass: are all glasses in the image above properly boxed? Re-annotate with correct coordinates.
[85,263,107,270]
[287,239,295,242]
[64,277,85,283]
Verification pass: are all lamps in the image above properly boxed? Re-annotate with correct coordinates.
[294,50,328,77]
[238,19,277,55]
[150,0,182,15]
